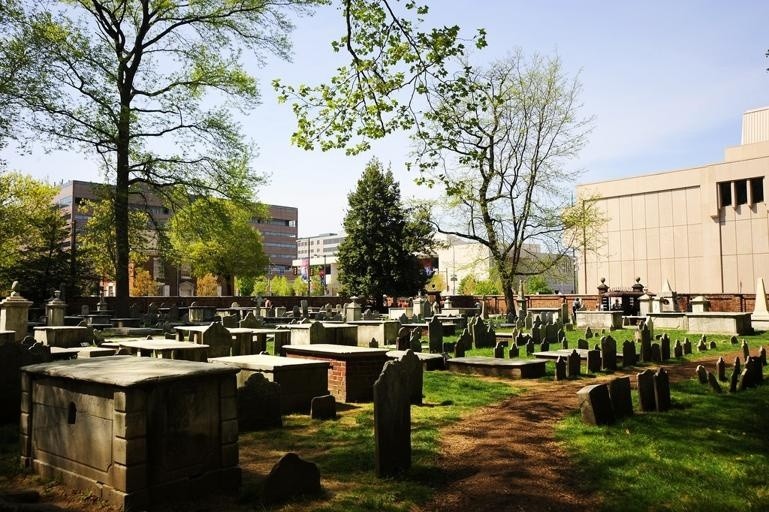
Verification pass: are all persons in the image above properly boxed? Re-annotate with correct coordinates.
[382,297,445,317]
[613,299,619,310]
[264,296,272,311]
[474,299,482,309]
[572,298,582,323]
